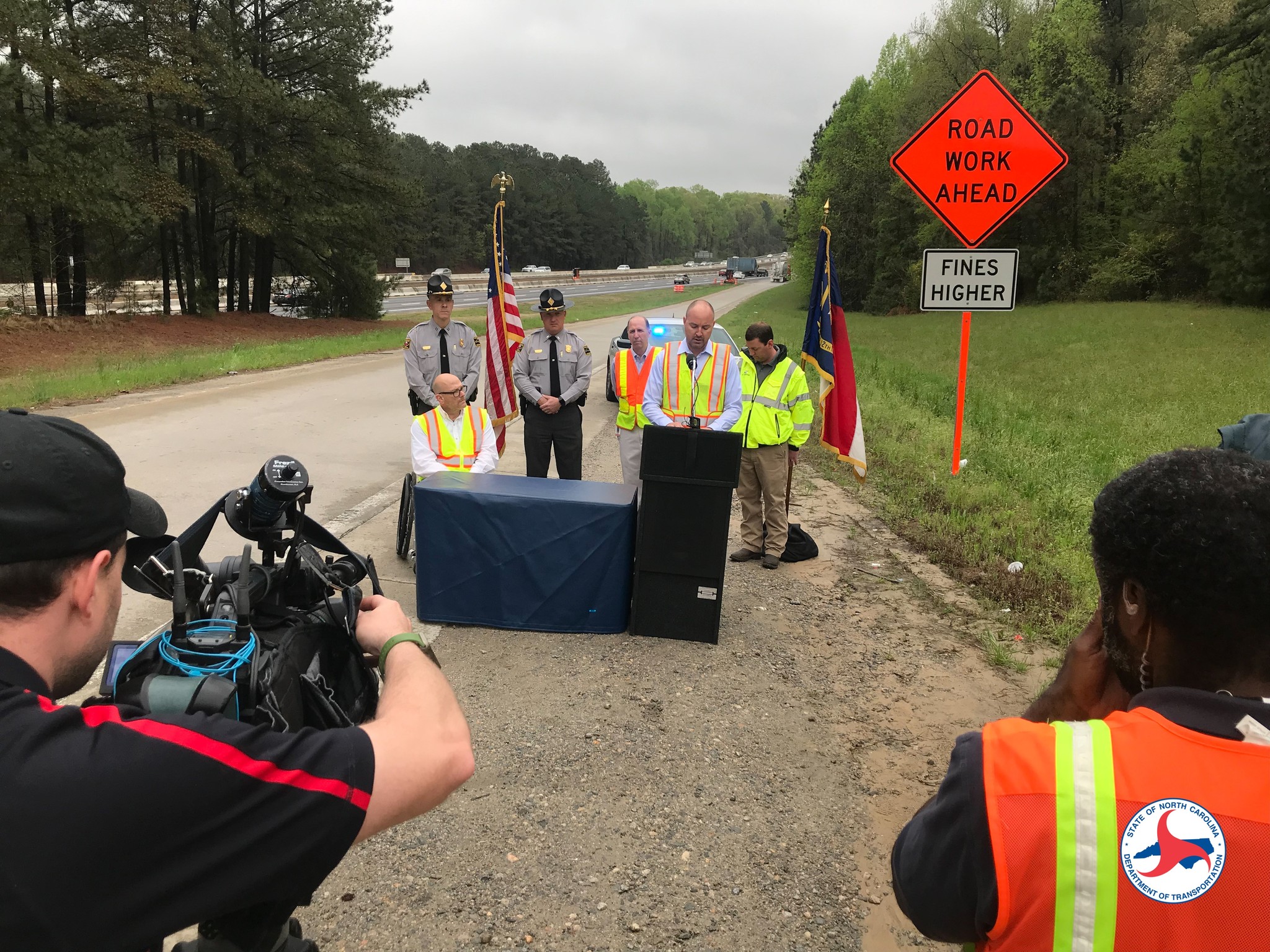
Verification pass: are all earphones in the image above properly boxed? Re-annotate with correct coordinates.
[1123,600,1138,614]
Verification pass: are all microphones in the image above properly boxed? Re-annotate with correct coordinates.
[330,555,367,587]
[686,354,697,370]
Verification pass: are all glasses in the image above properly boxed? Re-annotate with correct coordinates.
[439,385,467,397]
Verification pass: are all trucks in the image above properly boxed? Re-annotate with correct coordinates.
[726,257,759,276]
[770,261,791,282]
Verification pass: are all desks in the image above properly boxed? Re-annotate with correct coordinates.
[412,471,638,634]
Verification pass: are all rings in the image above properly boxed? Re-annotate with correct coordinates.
[791,460,794,461]
[546,410,547,413]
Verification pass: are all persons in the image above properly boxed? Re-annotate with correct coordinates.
[610,315,664,510]
[890,445,1270,952]
[410,373,499,483]
[0,409,476,952]
[512,289,593,481]
[641,300,743,432]
[403,274,483,415]
[729,322,814,568]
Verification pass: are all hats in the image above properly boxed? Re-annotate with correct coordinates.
[0,407,168,564]
[530,288,575,313]
[416,274,464,296]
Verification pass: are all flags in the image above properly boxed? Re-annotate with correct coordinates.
[801,225,867,484]
[484,201,526,459]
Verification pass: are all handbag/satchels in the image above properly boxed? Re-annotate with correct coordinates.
[761,521,819,562]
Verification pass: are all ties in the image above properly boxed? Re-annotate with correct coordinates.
[547,336,561,398]
[439,329,450,374]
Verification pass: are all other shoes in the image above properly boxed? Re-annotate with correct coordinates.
[762,554,780,568]
[729,547,760,561]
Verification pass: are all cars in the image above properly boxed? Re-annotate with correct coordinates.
[685,261,715,266]
[535,267,551,273]
[767,254,773,258]
[719,269,727,276]
[480,268,490,273]
[720,260,728,264]
[781,252,788,257]
[606,313,743,401]
[271,288,310,308]
[617,265,630,269]
[733,271,746,279]
[431,268,451,277]
[674,274,690,284]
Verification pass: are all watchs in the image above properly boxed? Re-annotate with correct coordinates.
[377,632,442,683]
[558,396,566,407]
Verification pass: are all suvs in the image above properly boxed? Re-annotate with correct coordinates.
[521,265,537,272]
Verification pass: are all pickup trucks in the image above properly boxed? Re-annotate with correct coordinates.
[757,269,769,277]
